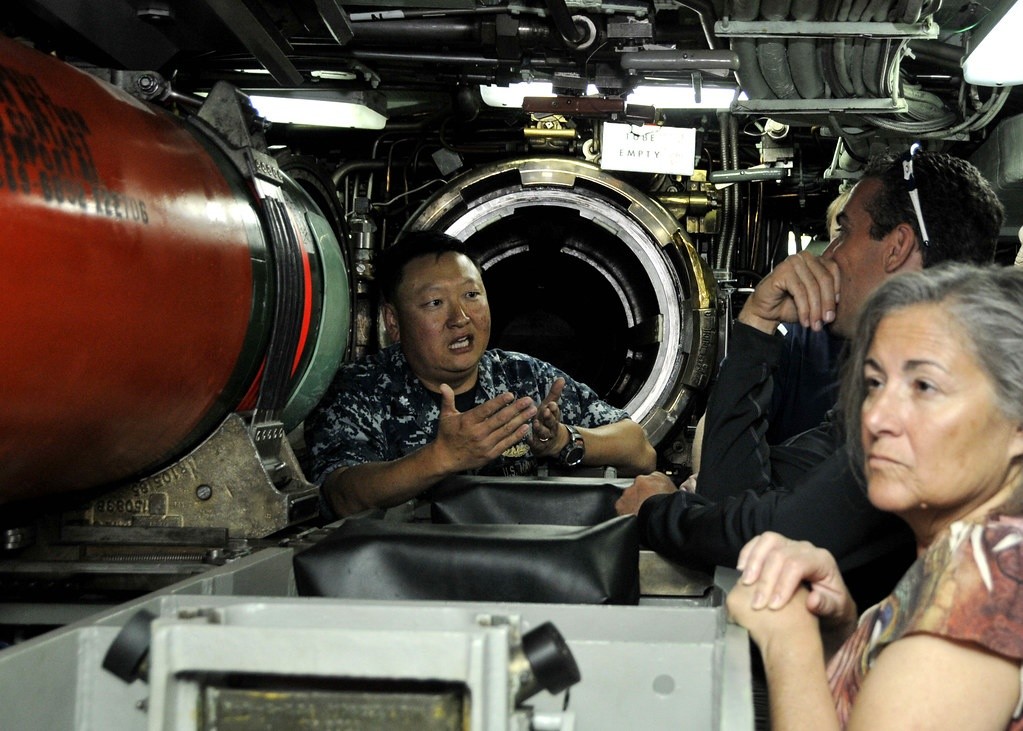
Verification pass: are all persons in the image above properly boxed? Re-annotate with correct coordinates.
[303,228,658,520]
[613,147,1007,618]
[723,259,1023,731]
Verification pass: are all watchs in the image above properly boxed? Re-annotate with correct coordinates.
[558,424,586,467]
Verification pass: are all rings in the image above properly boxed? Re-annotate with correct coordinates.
[540,430,554,442]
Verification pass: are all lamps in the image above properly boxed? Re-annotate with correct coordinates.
[194,89,390,130]
[480,77,748,110]
[963,0,1023,86]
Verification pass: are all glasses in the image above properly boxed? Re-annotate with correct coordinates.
[891,143,931,249]
